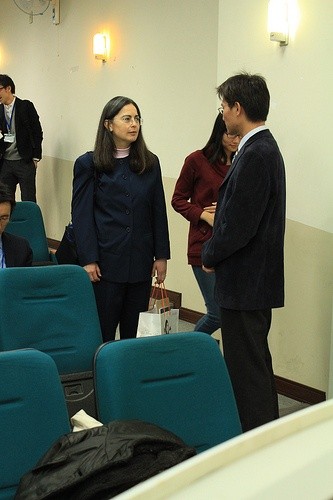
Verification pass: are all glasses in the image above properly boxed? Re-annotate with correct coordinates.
[223,130,243,139]
[112,116,143,126]
[217,103,232,115]
[0,85,7,92]
[0,215,12,224]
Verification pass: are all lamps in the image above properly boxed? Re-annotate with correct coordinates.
[270,0,297,45]
[92,31,108,63]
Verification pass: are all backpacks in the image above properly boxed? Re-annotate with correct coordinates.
[11,409,197,500]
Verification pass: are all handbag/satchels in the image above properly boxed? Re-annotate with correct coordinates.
[54,224,79,266]
[135,280,179,337]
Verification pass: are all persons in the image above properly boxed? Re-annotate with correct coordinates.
[170,110,243,336]
[71,96,170,343]
[0,74,43,269]
[200,73,286,432]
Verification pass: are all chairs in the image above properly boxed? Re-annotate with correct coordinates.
[0,347,70,500]
[3,201,50,267]
[93,331,243,455]
[0,264,103,376]
